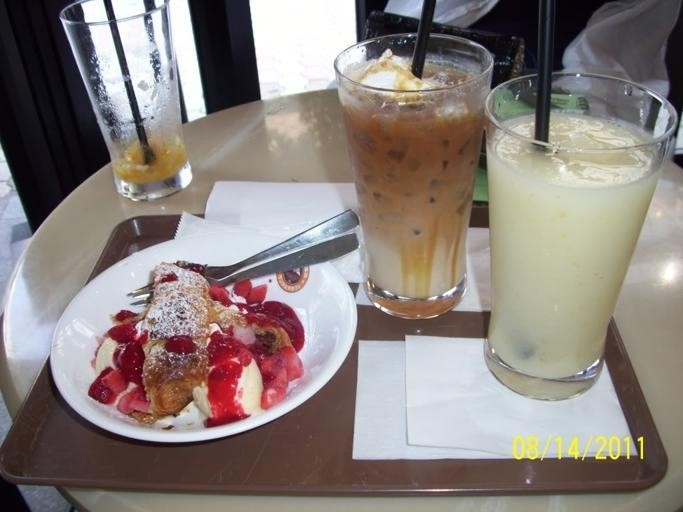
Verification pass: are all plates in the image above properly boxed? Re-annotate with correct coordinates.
[49,230,356,442]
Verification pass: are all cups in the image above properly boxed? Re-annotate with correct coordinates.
[482,73,678,402]
[58,0,193,203]
[333,33,496,321]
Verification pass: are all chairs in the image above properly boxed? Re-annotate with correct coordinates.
[361,10,526,100]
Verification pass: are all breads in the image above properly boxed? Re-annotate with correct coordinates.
[139,262,213,420]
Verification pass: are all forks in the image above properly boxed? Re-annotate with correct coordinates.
[129,209,358,304]
